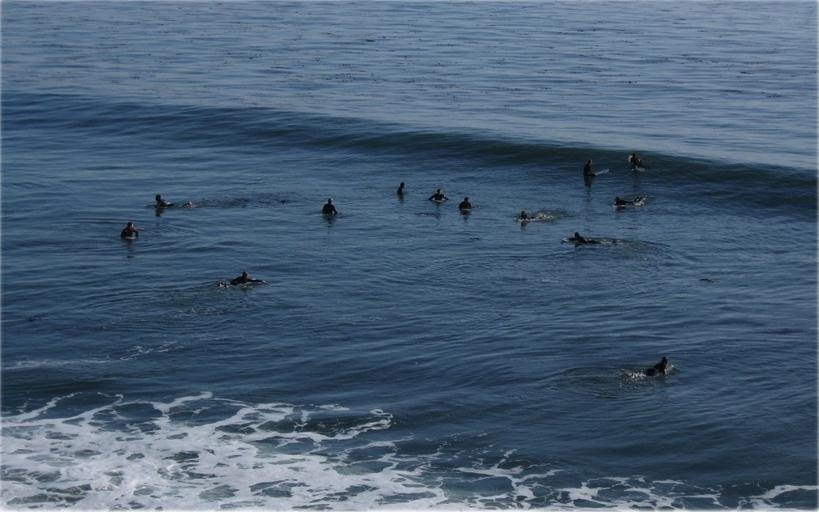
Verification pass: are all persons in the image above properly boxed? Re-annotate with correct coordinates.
[584,176,593,187]
[120,222,139,239]
[429,188,472,211]
[322,197,338,214]
[645,356,669,377]
[156,193,192,208]
[216,271,265,287]
[520,210,544,220]
[569,232,620,244]
[584,153,646,177]
[615,196,642,205]
[397,182,406,194]
